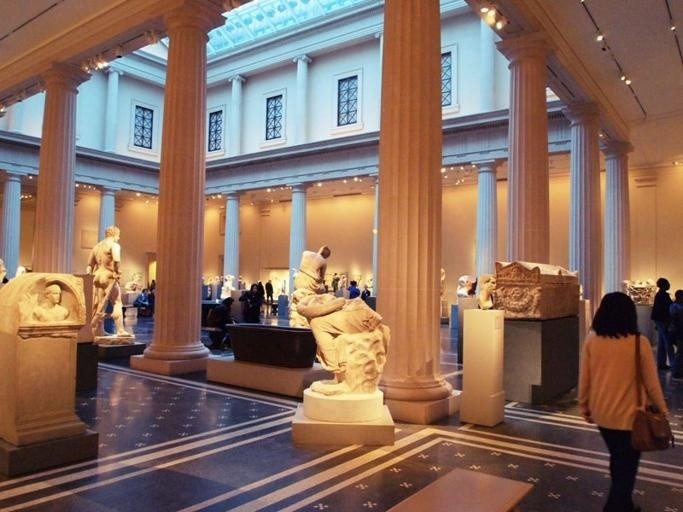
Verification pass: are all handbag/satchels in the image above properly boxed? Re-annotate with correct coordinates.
[629,409,675,452]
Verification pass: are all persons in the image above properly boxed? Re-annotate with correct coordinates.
[257,281,264,302]
[265,279,274,302]
[31,283,69,322]
[361,283,371,301]
[206,296,236,351]
[84,223,132,338]
[330,272,340,292]
[340,275,346,288]
[294,243,331,295]
[475,272,497,311]
[668,289,682,380]
[132,288,151,318]
[651,277,674,371]
[206,274,243,300]
[150,278,155,289]
[238,283,264,323]
[287,241,382,384]
[277,286,289,317]
[576,289,674,512]
[346,280,360,300]
[147,287,154,311]
[455,275,472,297]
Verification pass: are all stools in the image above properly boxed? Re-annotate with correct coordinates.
[201,326,224,350]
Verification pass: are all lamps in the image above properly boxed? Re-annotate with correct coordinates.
[480,0,508,30]
